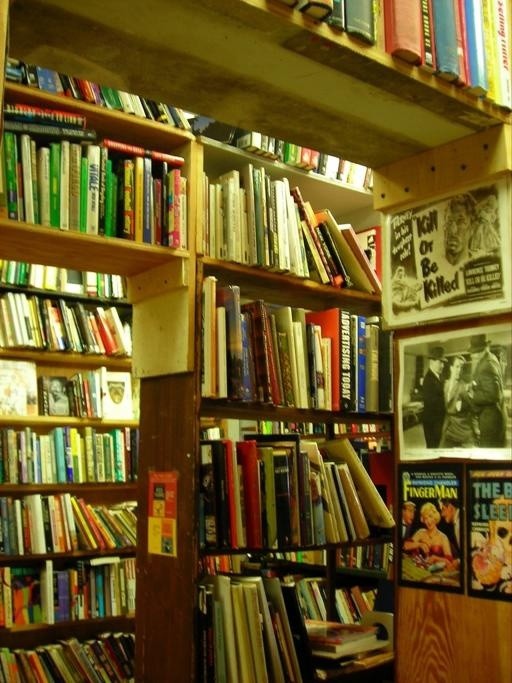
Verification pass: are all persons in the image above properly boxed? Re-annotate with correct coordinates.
[390,192,512,597]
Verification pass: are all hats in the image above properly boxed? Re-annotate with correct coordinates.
[426,347,448,363]
[467,335,491,352]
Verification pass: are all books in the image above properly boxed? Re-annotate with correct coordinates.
[278,0,512,111]
[3,56,396,683]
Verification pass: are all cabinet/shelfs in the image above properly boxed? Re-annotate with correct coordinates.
[0,0,512,683]
